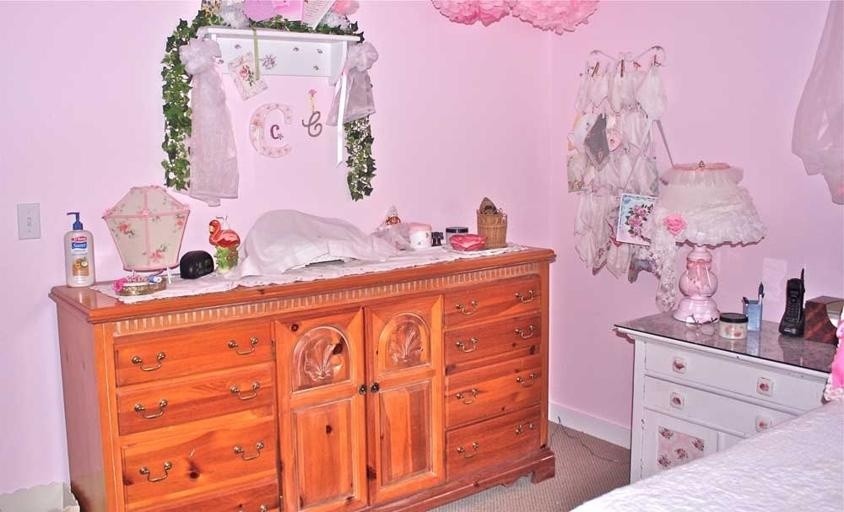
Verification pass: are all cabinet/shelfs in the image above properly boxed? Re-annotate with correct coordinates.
[617,327,831,483]
[48,247,557,512]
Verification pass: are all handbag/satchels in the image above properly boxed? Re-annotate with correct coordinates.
[616,193,658,246]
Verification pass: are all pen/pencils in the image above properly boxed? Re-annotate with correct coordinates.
[759,282,763,305]
[743,297,749,307]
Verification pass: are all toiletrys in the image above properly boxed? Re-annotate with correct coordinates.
[446,227,469,246]
[408,224,432,249]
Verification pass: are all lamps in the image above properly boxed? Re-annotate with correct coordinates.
[643,161,769,324]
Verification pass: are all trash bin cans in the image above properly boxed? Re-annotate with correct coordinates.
[0,481,81,512]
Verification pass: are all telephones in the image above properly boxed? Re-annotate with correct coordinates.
[779,268,805,336]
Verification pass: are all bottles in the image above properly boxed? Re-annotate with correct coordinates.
[446,227,468,248]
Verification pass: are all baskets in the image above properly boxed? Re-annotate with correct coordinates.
[476,208,508,249]
